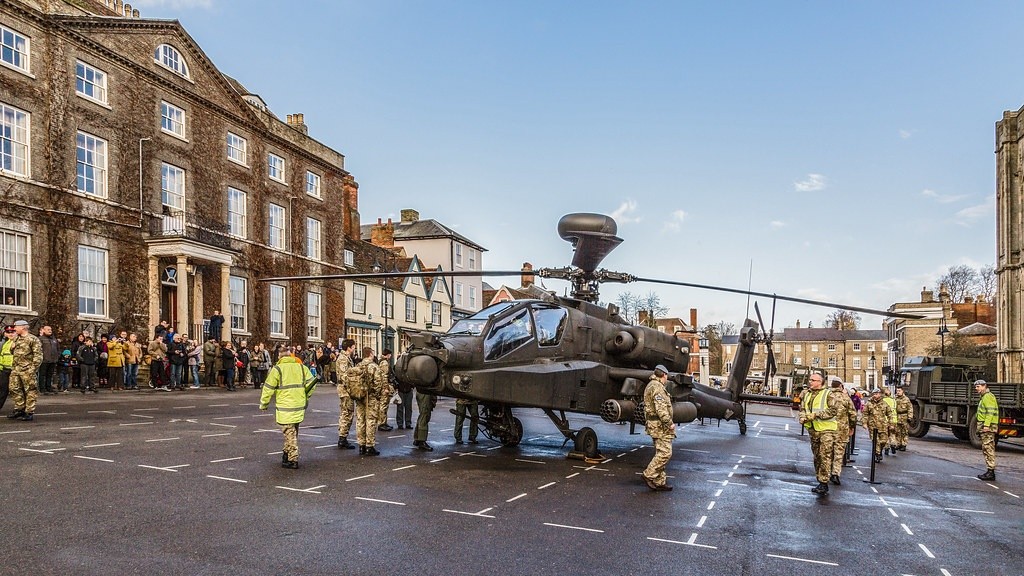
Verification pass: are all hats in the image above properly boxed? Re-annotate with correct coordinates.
[655,365,668,373]
[4,320,29,333]
[208,335,215,340]
[833,377,842,384]
[974,380,986,385]
[872,388,881,394]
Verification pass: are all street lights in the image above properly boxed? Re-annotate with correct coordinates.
[371,245,401,347]
[935,317,950,356]
[869,351,878,390]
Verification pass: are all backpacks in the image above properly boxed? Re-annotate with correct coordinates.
[344,362,376,401]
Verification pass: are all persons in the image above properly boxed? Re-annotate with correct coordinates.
[355,347,383,456]
[6,295,13,305]
[378,349,414,432]
[210,310,224,341]
[641,365,677,490]
[714,378,721,390]
[0,320,43,421]
[413,392,438,451]
[894,386,913,451]
[974,380,999,481]
[259,351,317,470]
[861,387,898,464]
[799,372,861,494]
[510,303,550,342]
[336,339,356,450]
[34,320,378,394]
[454,399,479,443]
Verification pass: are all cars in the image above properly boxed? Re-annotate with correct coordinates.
[849,386,869,397]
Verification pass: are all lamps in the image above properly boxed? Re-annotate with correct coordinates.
[368,314,372,319]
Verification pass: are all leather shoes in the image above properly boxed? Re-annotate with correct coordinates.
[978,469,995,481]
[282,462,298,468]
[338,438,355,449]
[642,475,672,491]
[359,445,380,455]
[378,424,479,451]
[282,453,288,462]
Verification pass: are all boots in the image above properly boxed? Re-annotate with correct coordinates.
[217,375,226,388]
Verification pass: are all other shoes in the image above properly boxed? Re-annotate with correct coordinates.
[190,384,200,388]
[875,445,906,463]
[205,383,209,386]
[43,384,124,396]
[830,475,841,485]
[812,483,828,494]
[6,410,34,421]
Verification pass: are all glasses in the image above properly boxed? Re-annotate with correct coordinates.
[175,338,181,340]
[809,379,820,382]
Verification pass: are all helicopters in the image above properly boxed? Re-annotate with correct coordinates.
[254,211,928,459]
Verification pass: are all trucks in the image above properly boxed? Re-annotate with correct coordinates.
[893,355,1024,450]
[776,374,846,397]
